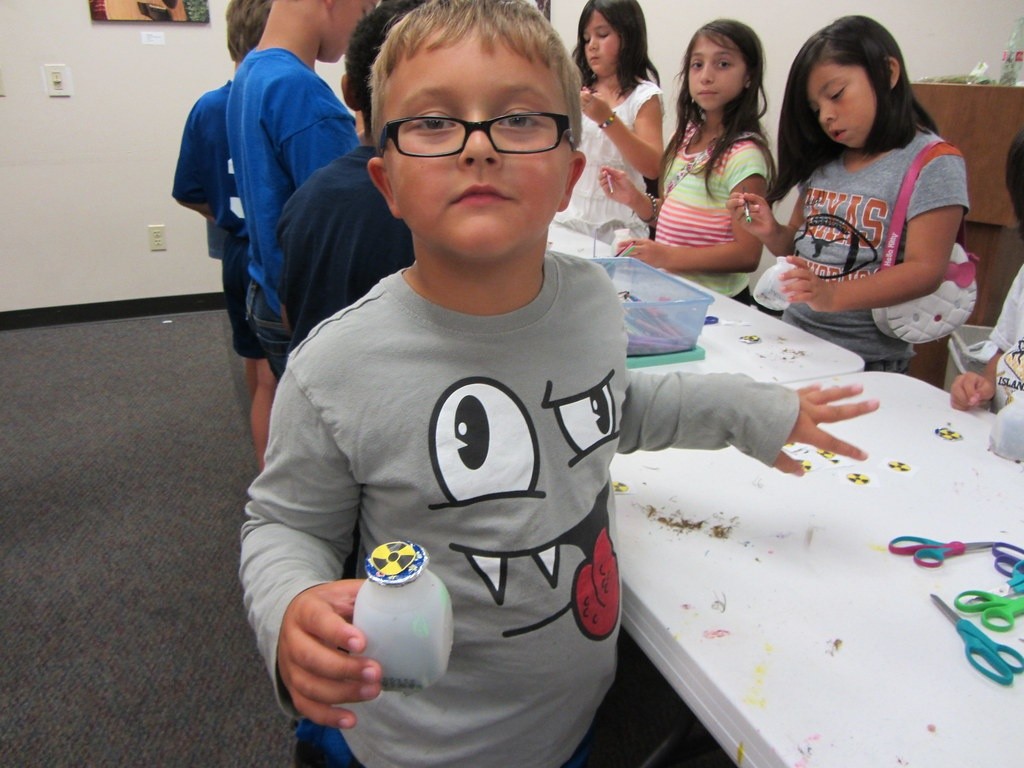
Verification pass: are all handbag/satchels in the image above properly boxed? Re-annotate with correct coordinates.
[872,140,981,346]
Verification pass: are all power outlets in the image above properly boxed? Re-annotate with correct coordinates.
[149,224,166,251]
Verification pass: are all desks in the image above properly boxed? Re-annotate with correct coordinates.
[609,372,1024,767]
[545,221,866,383]
[904,82,1024,389]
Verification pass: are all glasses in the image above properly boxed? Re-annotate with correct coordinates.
[379,111,576,158]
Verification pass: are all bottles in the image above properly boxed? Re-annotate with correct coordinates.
[753,256,799,311]
[350,541,454,692]
[989,391,1024,461]
[610,229,631,257]
[999,18,1024,85]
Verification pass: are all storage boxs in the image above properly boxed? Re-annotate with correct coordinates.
[584,256,715,355]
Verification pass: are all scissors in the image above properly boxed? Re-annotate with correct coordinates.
[954,590,1024,632]
[930,594,1024,685]
[889,536,996,567]
[993,542,1024,578]
[965,560,1024,604]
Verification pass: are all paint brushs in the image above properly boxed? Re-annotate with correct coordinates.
[742,186,751,222]
[623,293,695,353]
[606,171,614,194]
[590,90,598,94]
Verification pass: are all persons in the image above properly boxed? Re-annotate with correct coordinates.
[949,128,1024,416]
[171,0,426,478]
[552,0,665,248]
[599,19,776,307]
[726,16,969,373]
[239,0,879,768]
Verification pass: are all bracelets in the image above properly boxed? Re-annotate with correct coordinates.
[639,193,657,222]
[598,112,616,128]
